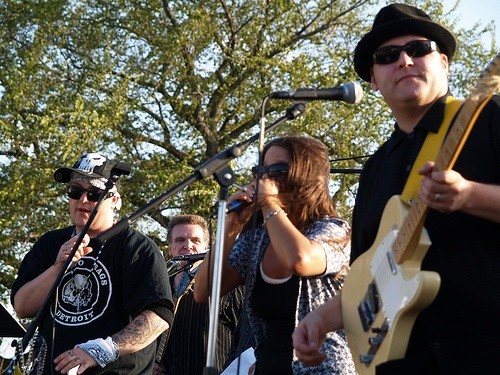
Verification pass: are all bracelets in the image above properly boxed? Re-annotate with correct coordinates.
[262,210,288,227]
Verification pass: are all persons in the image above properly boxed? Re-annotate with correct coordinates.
[11,152,174,375]
[292,4,500,375]
[156,214,255,375]
[193,137,359,375]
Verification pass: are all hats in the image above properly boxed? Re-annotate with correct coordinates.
[353,3,456,83]
[53,152,120,192]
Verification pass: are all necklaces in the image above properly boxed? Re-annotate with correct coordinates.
[71,226,106,311]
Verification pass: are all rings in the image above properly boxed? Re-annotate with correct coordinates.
[434,193,440,202]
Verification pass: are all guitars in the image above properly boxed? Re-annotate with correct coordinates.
[338,52,500,375]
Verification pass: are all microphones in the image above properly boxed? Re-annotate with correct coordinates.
[213,194,256,220]
[269,82,364,104]
[171,254,207,262]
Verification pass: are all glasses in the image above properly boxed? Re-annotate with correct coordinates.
[370,40,441,65]
[252,162,290,180]
[65,185,114,202]
